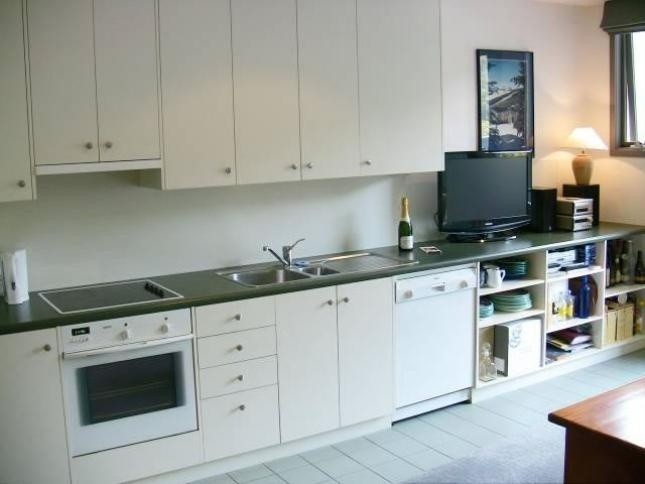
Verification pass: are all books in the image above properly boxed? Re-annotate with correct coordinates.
[547,330,593,351]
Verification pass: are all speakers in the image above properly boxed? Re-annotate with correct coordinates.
[533,187,558,234]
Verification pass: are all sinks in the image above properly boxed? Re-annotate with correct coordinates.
[294,263,342,277]
[215,265,313,286]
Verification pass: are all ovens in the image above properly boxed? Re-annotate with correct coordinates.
[57,307,199,457]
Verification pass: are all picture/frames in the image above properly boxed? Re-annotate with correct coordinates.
[476,50,534,157]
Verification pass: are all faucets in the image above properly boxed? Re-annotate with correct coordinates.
[262,237,306,267]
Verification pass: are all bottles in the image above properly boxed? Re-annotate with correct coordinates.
[620,241,628,274]
[608,240,617,286]
[578,275,590,318]
[627,241,635,284]
[606,251,611,288]
[568,290,573,320]
[615,255,621,285]
[398,197,413,253]
[479,343,497,381]
[634,250,645,284]
[558,291,566,322]
[621,254,630,283]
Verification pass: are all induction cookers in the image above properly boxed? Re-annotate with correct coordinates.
[38,279,184,314]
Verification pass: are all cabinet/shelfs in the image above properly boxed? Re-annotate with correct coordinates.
[1,292,72,484]
[472,221,645,405]
[277,251,393,459]
[359,1,445,177]
[392,246,480,424]
[138,0,235,190]
[25,1,163,175]
[194,261,281,481]
[0,0,36,204]
[230,1,358,186]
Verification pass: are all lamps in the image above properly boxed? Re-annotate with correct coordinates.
[560,127,608,185]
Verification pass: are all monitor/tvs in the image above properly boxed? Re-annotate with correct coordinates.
[435,149,533,244]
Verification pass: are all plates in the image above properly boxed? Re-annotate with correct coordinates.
[489,289,533,312]
[496,257,530,280]
[480,298,494,318]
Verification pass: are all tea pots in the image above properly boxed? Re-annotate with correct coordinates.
[486,268,505,288]
